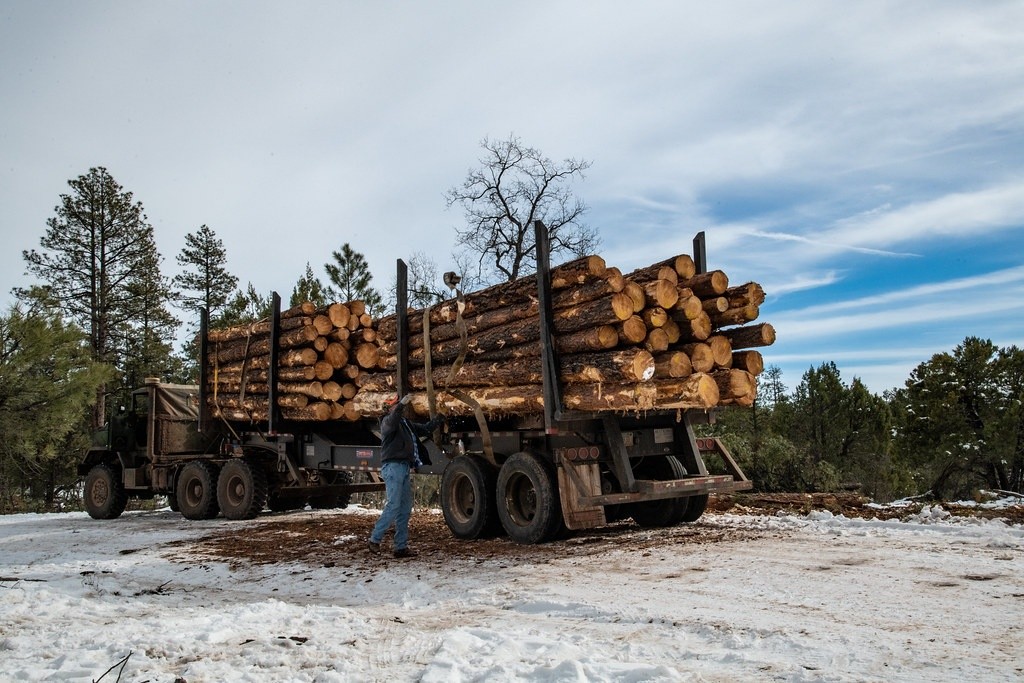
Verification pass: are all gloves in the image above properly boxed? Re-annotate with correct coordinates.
[401,393,415,405]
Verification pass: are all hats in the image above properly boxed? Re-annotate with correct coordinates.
[382,392,398,413]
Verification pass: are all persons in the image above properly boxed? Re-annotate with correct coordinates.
[367,394,446,558]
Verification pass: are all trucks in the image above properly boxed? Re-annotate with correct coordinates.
[76,221,754,544]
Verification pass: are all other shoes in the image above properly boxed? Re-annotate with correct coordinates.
[393,547,417,558]
[367,541,381,554]
